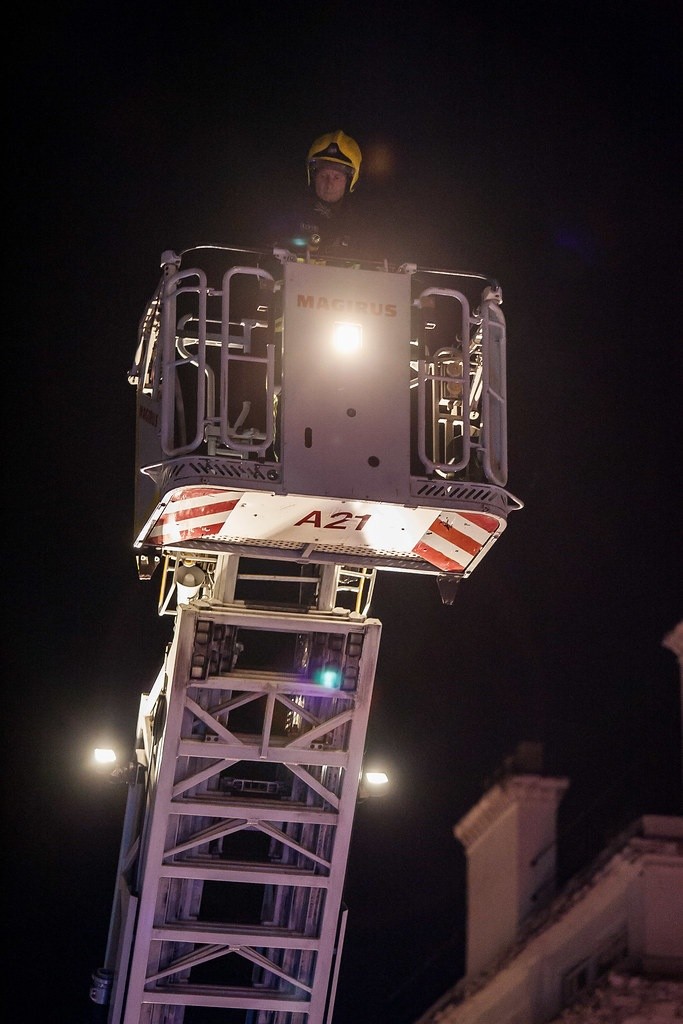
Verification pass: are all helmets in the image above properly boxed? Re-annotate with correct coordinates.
[306,130,362,194]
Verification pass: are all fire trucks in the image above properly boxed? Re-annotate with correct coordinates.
[91,235,529,1024]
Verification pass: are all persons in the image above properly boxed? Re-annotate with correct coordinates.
[256,131,432,479]
